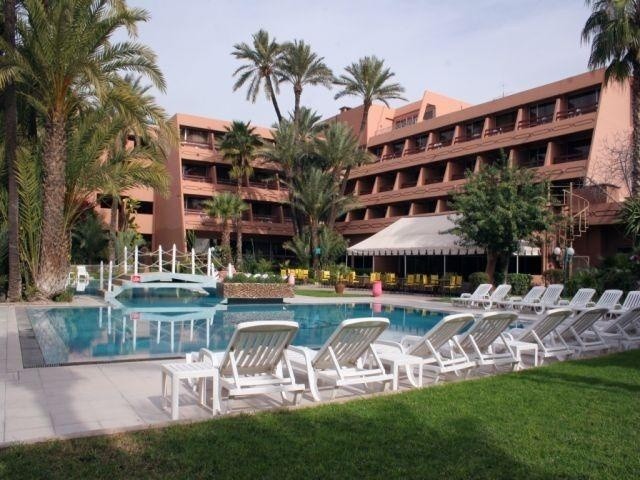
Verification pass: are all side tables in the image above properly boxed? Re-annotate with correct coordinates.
[160,361,221,423]
[366,354,424,389]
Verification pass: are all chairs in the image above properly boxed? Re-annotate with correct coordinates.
[184,319,305,417]
[279,268,465,293]
[282,316,396,404]
[451,281,640,381]
[374,312,474,382]
[398,310,520,371]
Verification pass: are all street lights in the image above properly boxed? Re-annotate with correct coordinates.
[551,246,575,297]
